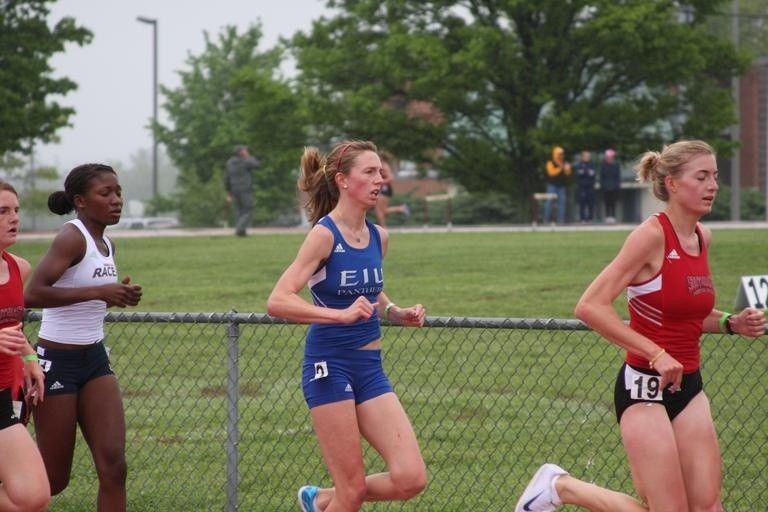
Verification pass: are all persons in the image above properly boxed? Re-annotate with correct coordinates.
[570,149,598,225]
[0,179,53,511]
[515,138,768,512]
[223,142,261,237]
[265,138,428,511]
[21,164,144,511]
[541,145,573,227]
[373,149,411,230]
[593,149,621,225]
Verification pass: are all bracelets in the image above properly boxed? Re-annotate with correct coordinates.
[725,314,739,334]
[383,301,394,323]
[647,346,669,364]
[719,310,732,335]
[21,353,39,363]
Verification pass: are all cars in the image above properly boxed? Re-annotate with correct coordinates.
[103,216,179,232]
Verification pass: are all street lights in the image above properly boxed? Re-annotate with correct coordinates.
[137,14,161,202]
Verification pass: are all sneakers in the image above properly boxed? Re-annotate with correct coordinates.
[515,464,567,512]
[297,485,320,512]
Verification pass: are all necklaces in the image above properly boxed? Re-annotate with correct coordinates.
[335,210,368,245]
